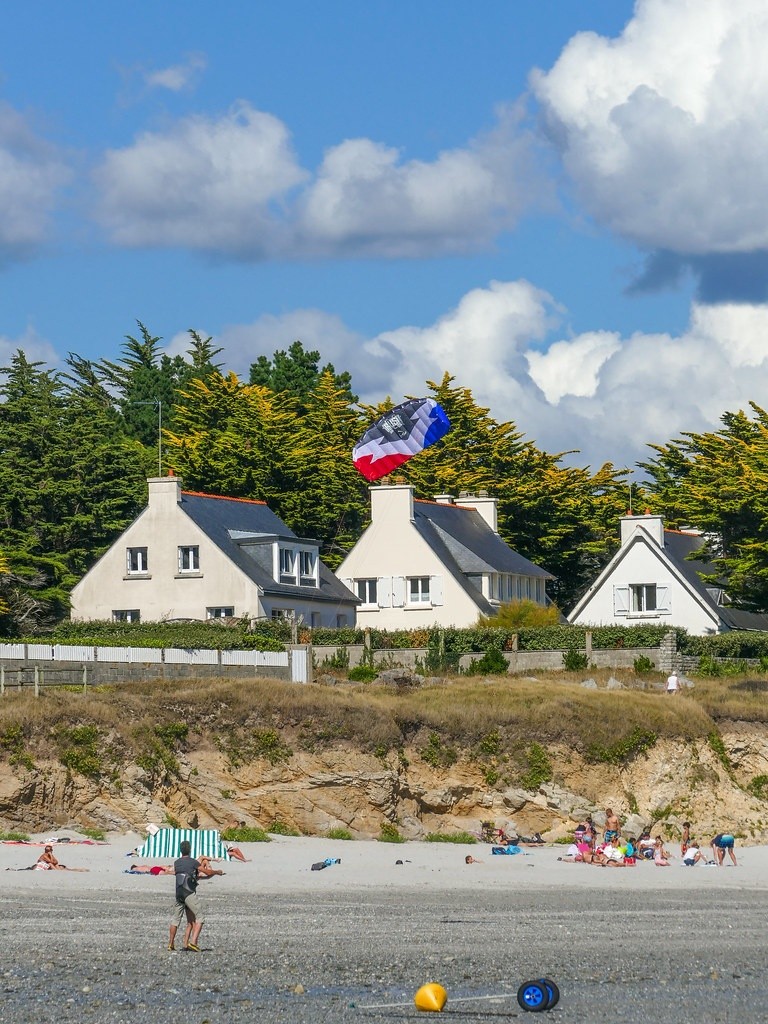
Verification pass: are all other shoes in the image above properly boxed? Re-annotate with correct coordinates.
[168,943,174,950]
[182,947,188,950]
[188,942,199,952]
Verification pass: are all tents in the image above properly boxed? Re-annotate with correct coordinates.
[138,830,229,861]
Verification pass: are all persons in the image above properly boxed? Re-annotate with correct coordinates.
[4,842,250,877]
[665,672,681,696]
[167,840,223,952]
[466,855,476,864]
[557,809,738,866]
